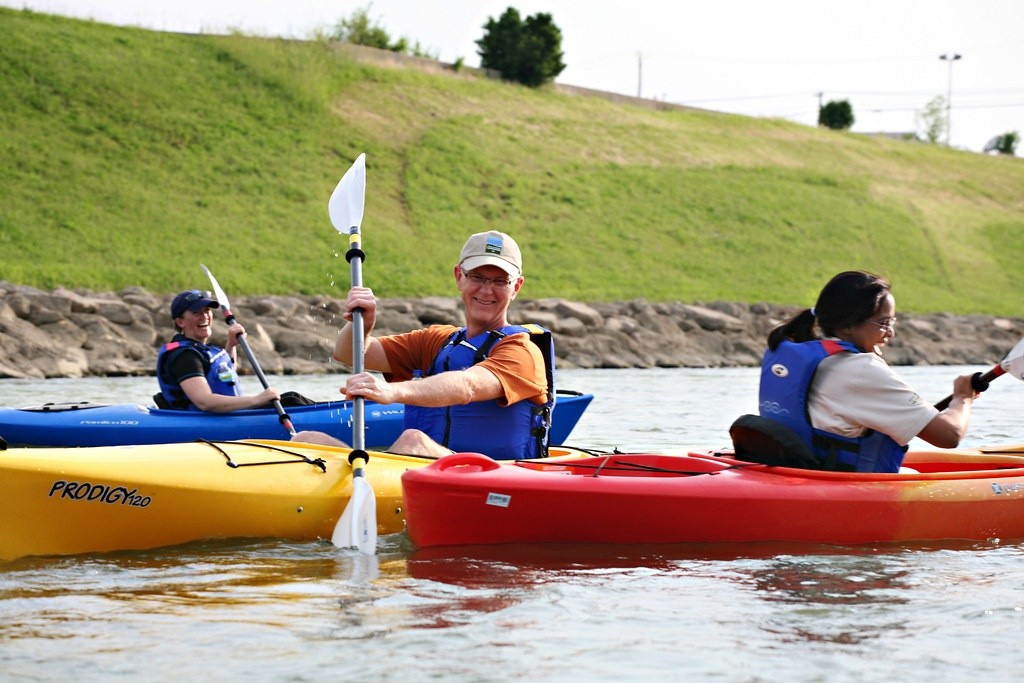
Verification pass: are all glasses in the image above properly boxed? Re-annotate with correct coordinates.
[867,318,897,332]
[184,291,212,301]
[462,269,517,288]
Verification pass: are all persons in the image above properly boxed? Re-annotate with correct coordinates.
[759,270,983,474]
[334,231,556,460]
[157,290,316,412]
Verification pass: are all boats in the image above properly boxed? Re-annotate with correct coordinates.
[401,439,1023,548]
[0,438,612,564]
[0,394,592,453]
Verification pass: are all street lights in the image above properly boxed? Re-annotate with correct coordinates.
[939,54,963,143]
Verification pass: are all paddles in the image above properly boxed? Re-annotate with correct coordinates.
[196,258,302,438]
[325,151,381,562]
[934,335,1024,412]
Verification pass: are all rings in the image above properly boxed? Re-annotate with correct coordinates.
[361,382,366,388]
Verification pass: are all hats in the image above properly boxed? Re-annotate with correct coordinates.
[170,292,220,319]
[459,230,523,278]
